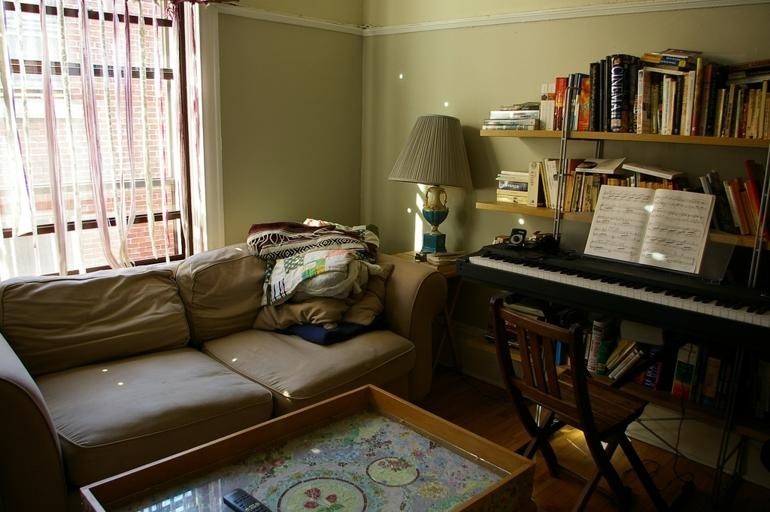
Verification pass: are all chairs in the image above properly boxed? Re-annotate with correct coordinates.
[490,294,668,511]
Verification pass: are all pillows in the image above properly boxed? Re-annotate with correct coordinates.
[0,267,191,377]
[175,246,266,344]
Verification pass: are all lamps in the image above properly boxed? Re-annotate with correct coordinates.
[389,115,473,261]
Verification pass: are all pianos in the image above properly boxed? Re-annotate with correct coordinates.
[457,243,770,350]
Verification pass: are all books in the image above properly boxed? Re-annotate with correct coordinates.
[583,181,716,275]
[481,46,770,235]
[482,284,770,424]
[426,250,468,267]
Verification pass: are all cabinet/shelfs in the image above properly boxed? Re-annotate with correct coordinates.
[476,130,770,282]
[622,285,626,287]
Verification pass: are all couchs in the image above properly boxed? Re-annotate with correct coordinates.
[1,243,449,512]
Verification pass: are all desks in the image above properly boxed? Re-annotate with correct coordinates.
[393,251,463,374]
